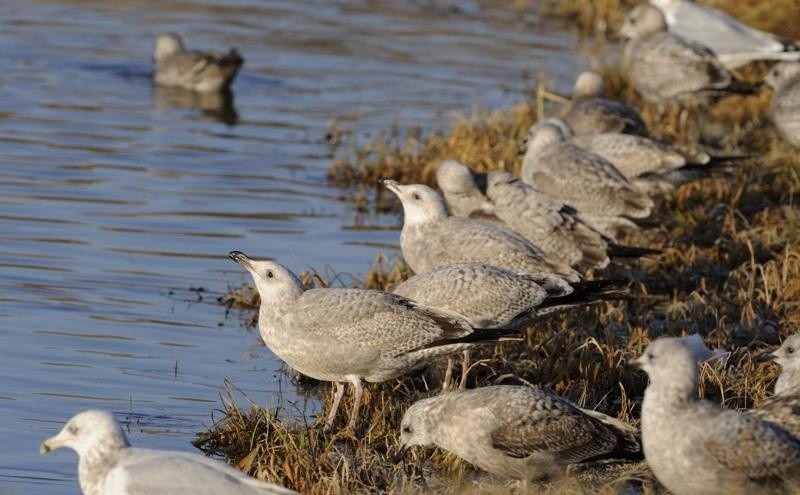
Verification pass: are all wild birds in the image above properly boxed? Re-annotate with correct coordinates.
[624,334,800,495]
[40,409,304,495]
[228,251,527,433]
[394,385,645,482]
[378,71,757,353]
[152,33,243,91]
[604,0,800,151]
